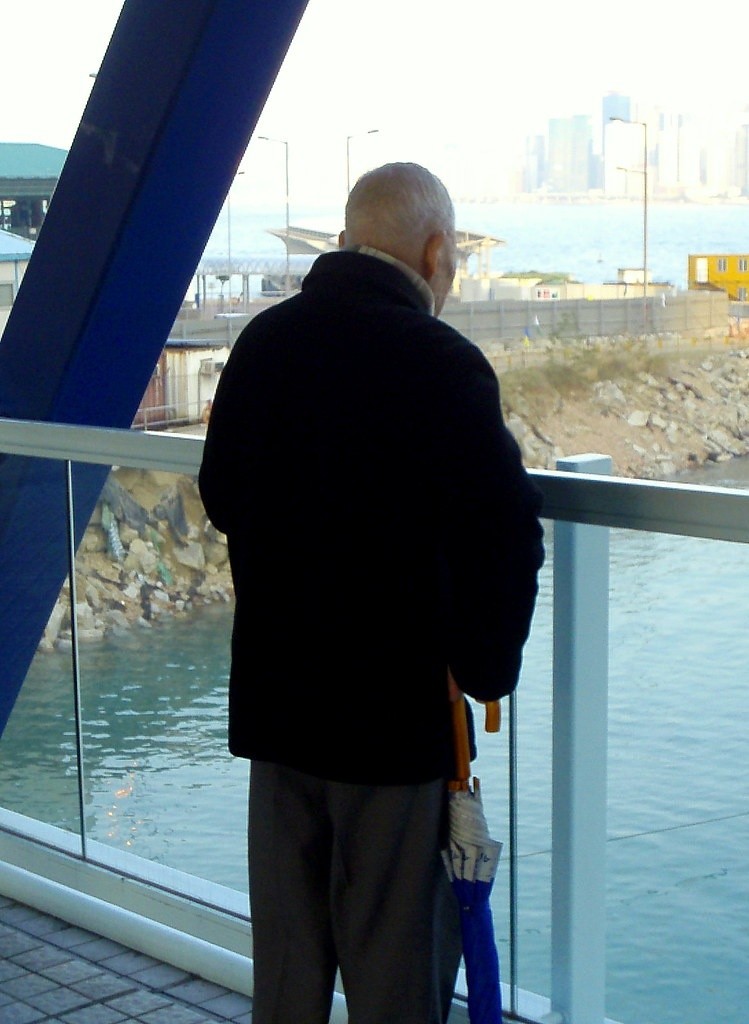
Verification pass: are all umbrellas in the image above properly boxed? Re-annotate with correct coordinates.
[439,675,504,1024]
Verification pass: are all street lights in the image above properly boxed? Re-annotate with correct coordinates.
[258,137,290,287]
[609,116,648,302]
[346,130,378,195]
[227,172,244,315]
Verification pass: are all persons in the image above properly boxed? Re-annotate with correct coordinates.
[198,162,544,1024]
